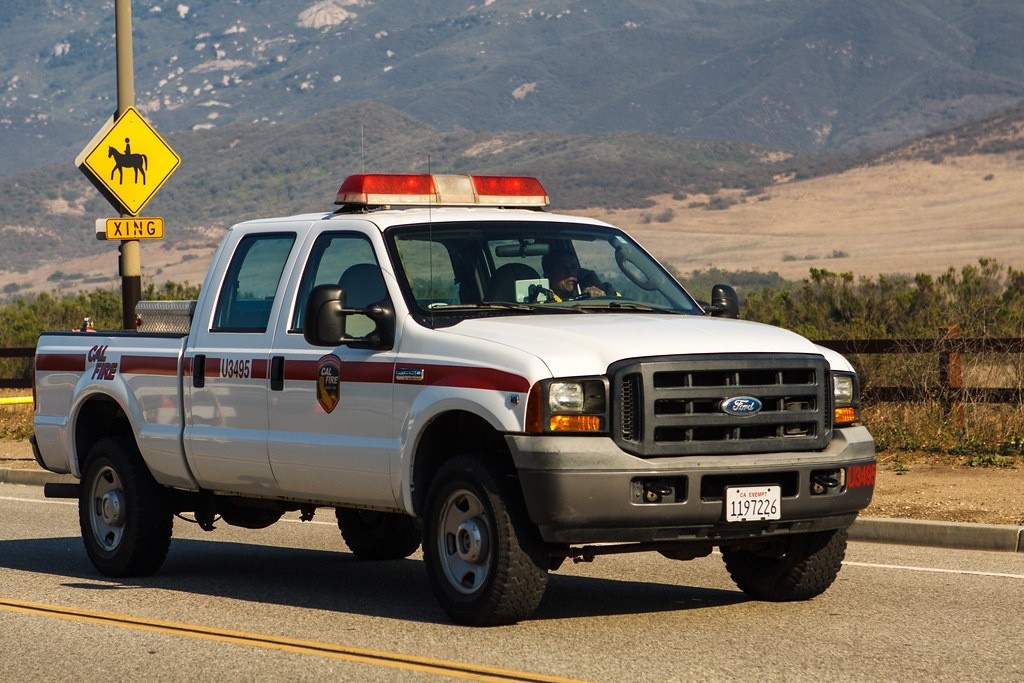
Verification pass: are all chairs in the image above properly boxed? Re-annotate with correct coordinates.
[491,261,544,302]
[338,263,388,309]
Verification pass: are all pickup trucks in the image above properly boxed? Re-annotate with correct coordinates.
[28,172,878,626]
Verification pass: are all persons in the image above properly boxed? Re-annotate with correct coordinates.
[531,244,624,304]
